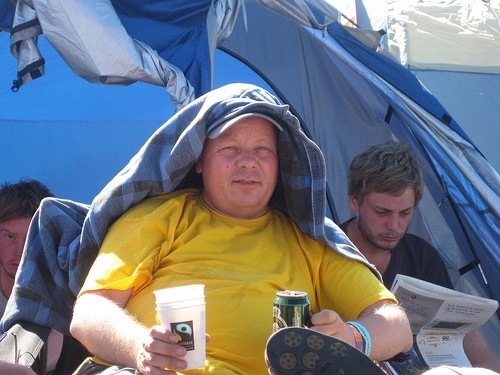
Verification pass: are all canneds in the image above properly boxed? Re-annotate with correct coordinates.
[272,290,312,332]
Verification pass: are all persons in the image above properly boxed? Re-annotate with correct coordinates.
[264,139,500,375]
[0,178,65,321]
[69,81,413,375]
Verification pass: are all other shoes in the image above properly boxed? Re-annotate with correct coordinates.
[265,326,386,375]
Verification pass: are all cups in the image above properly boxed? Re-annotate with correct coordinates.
[153,283,206,370]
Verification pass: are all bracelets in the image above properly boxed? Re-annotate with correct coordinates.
[346,322,371,358]
